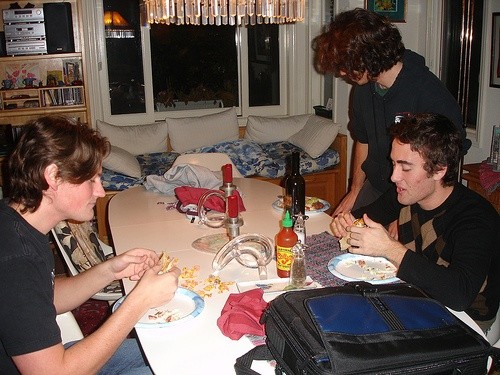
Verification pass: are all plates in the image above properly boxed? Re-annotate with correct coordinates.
[327,253,400,284]
[272,196,330,215]
[112,286,205,328]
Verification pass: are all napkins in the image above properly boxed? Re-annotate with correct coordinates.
[216,288,268,340]
[174,186,246,211]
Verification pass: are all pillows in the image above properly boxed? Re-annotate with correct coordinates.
[95,120,167,156]
[165,106,239,152]
[245,114,312,143]
[101,145,142,178]
[288,116,342,159]
[173,152,243,179]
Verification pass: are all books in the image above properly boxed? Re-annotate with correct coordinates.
[42,88,81,106]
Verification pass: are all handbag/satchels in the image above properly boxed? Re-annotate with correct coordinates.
[175,186,246,215]
[234,280,500,375]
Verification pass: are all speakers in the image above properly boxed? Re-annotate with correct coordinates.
[43,2,75,53]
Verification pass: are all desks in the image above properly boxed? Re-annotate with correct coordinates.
[108,178,405,375]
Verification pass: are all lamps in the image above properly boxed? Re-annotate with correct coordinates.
[104,0,135,38]
[139,0,305,25]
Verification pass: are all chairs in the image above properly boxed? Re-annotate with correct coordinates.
[50,219,126,345]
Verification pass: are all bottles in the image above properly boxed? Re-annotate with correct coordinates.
[284,151,305,221]
[289,243,306,289]
[276,210,298,278]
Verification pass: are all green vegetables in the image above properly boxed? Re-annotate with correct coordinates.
[307,196,318,205]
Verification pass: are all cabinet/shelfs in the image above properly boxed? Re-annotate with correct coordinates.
[461,163,500,214]
[0,52,92,129]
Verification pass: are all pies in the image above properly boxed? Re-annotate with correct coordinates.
[158,252,177,274]
[338,217,366,250]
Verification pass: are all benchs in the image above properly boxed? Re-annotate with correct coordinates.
[96,126,348,247]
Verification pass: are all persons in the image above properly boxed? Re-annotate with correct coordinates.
[0,115,181,375]
[311,7,460,240]
[330,112,500,335]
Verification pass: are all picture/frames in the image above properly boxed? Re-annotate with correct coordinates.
[364,0,407,23]
[489,12,500,88]
